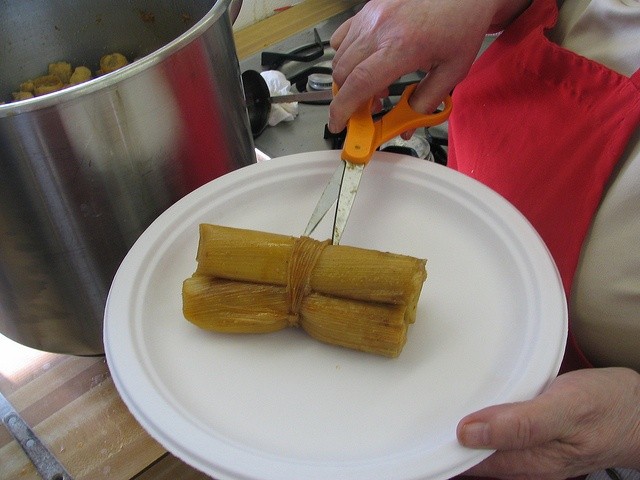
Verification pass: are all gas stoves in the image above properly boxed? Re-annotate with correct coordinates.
[237,2,502,166]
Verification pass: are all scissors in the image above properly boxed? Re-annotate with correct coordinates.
[304,81,452,246]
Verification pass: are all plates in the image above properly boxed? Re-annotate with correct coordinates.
[102,151,569,480]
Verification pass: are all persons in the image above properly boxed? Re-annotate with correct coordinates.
[327,0,640,480]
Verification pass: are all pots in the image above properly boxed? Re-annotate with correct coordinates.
[0,0,257,357]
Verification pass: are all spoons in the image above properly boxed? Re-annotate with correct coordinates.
[241,70,420,139]
